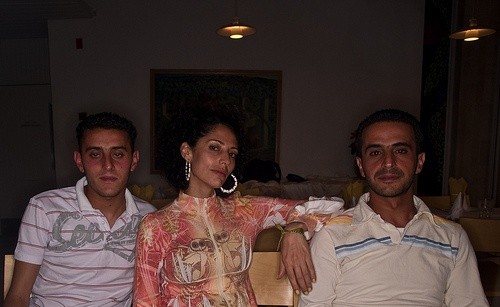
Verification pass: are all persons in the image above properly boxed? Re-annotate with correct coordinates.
[197,80,261,181]
[3,112,158,307]
[131,111,345,307]
[297,109,489,307]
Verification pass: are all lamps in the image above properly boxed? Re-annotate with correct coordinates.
[216,0,256,40]
[448,0,496,42]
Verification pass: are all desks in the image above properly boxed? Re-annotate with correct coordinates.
[434,206,500,255]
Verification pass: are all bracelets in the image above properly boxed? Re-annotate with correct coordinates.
[272,220,304,251]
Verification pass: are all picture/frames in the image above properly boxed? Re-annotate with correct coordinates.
[149,68,283,178]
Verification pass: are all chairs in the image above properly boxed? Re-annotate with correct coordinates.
[4,247,299,307]
